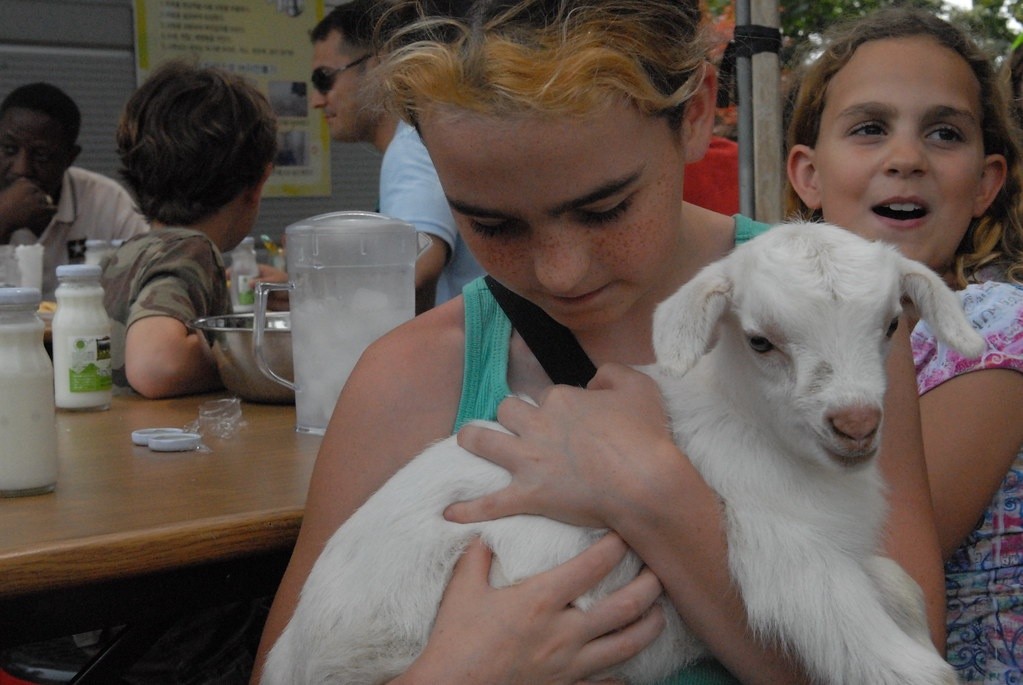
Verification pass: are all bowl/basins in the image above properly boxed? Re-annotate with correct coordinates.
[186,311,295,405]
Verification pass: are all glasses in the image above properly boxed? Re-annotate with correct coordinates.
[309,52,374,97]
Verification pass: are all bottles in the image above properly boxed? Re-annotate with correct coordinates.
[0,287,59,497]
[230,237,258,313]
[51,264,112,411]
[110,239,126,256]
[83,240,107,264]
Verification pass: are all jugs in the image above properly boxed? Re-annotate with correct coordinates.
[252,210,433,438]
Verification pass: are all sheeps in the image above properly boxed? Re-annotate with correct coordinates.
[262,217,988,684]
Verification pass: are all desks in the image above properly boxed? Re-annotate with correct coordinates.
[0,395,323,684]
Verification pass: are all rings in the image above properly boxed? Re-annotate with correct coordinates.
[45,194,53,205]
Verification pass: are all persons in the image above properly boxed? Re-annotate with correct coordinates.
[225,0,488,317]
[99,58,281,402]
[782,9,1022,685]
[251,0,949,685]
[0,82,151,293]
